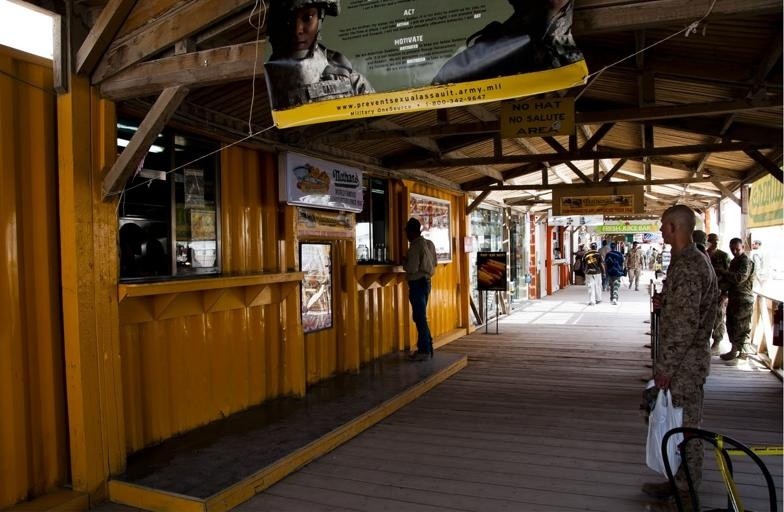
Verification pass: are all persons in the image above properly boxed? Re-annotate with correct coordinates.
[403,218,436,361]
[570,229,766,358]
[642,206,716,508]
[119,222,171,277]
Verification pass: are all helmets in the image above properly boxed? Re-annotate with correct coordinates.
[707,234,719,242]
[692,230,706,243]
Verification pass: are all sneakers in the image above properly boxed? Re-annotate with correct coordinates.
[643,491,701,512]
[407,350,429,360]
[641,480,678,500]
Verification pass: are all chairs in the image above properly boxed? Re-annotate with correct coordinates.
[661,426,778,511]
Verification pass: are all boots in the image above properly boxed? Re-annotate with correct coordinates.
[711,340,750,366]
